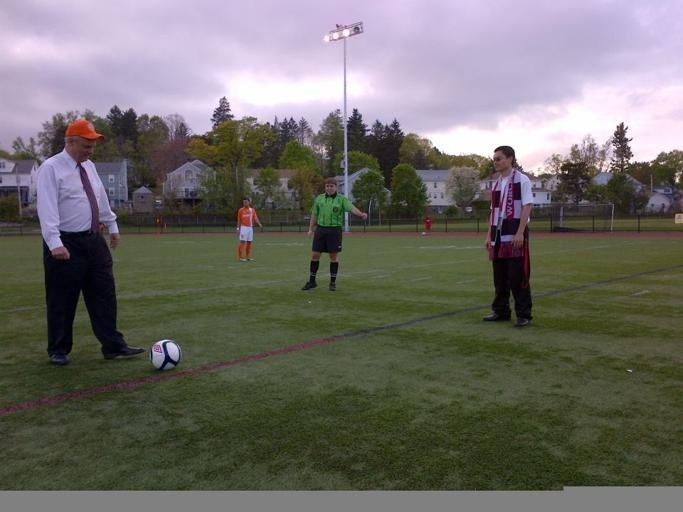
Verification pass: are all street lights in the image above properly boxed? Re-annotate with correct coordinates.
[324,22,364,229]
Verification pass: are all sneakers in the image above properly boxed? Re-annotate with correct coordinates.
[329,283,336,290]
[303,281,317,289]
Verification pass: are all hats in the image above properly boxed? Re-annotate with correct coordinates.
[66,119,104,140]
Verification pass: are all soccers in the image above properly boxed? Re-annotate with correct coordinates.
[422,232,425,236]
[149,340,181,370]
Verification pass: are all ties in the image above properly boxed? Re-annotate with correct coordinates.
[77,162,99,234]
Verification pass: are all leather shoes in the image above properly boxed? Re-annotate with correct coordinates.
[483,314,510,321]
[51,355,68,365]
[515,319,528,326]
[105,346,144,359]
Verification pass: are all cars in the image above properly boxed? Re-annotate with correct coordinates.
[463,207,471,214]
[155,197,162,206]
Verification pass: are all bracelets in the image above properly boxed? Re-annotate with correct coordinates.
[237,227,240,230]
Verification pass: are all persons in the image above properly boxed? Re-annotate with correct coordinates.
[483,145,535,326]
[301,176,368,290]
[424,214,433,233]
[35,119,146,365]
[235,198,263,262]
